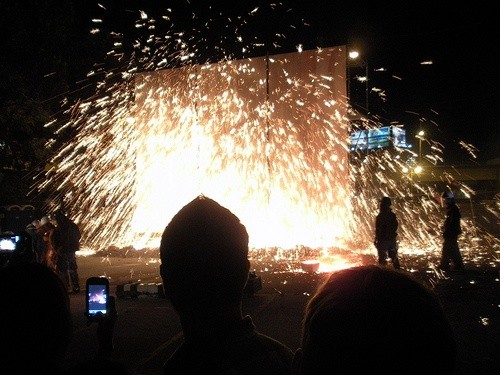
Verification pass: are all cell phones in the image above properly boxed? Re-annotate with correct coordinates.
[86,277,109,317]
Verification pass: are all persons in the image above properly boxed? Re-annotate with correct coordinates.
[0,205,81,293]
[437,193,465,281]
[289,265,461,375]
[133,198,294,375]
[374,197,400,269]
[0,261,118,375]
